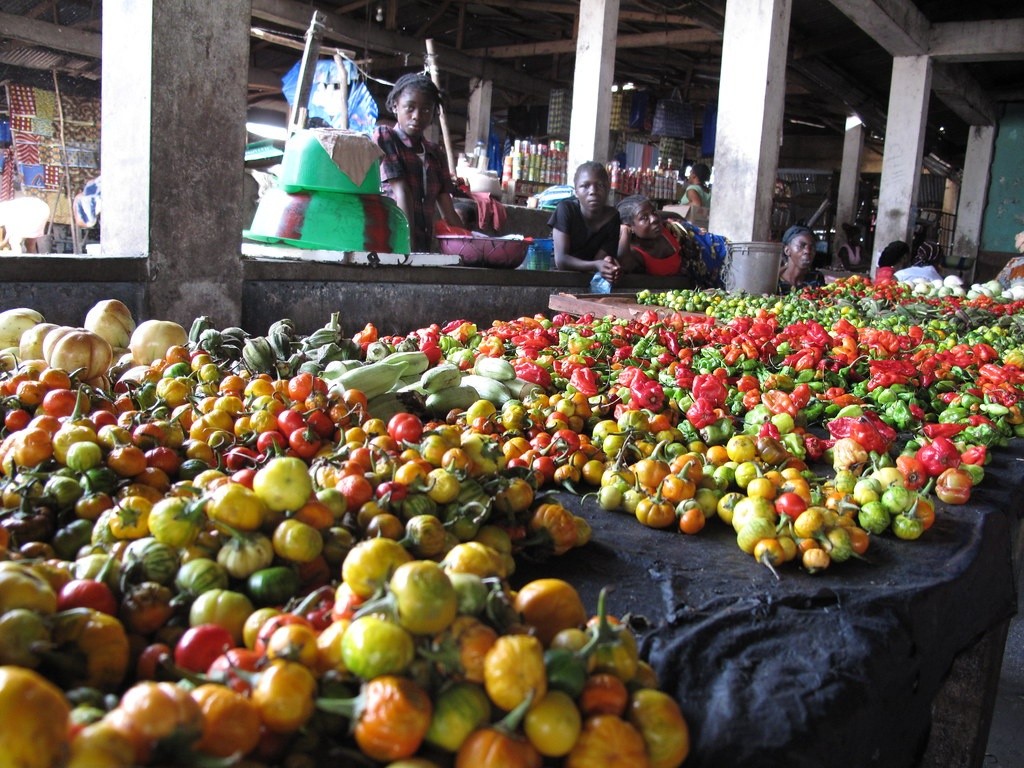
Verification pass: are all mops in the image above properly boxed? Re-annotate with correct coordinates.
[426,36,507,231]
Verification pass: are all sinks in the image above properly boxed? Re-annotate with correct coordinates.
[453,198,554,239]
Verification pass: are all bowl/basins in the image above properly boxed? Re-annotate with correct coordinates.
[278,127,382,197]
[436,234,532,269]
[240,181,411,258]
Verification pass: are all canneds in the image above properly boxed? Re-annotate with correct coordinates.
[501,136,682,200]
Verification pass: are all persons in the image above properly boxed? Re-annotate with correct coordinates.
[616,195,684,277]
[838,222,864,271]
[876,241,911,284]
[681,163,710,208]
[547,162,622,285]
[375,73,463,251]
[914,241,945,278]
[775,225,825,295]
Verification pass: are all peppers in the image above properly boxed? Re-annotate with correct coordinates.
[0,268,1024,768]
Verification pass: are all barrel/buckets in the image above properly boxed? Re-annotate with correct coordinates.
[525,238,555,270]
[720,241,783,300]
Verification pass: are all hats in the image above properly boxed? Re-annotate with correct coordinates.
[693,163,711,181]
